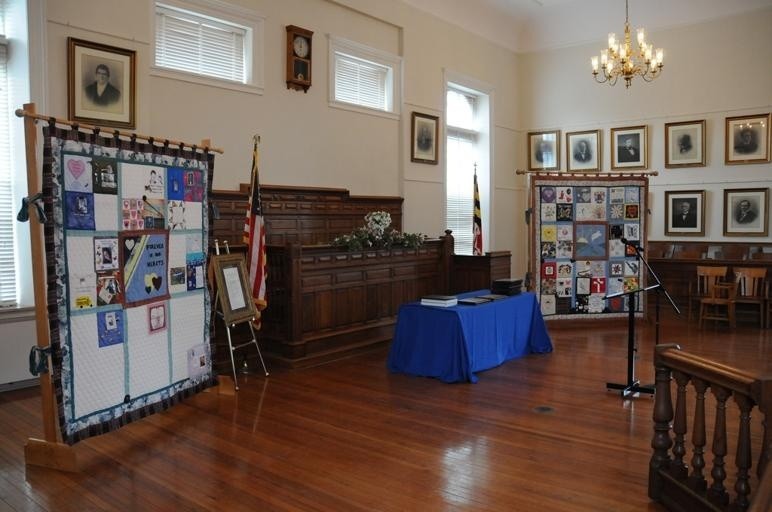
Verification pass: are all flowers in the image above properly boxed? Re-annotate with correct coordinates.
[331,210,423,253]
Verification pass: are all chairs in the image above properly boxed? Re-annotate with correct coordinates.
[698,282,737,328]
[731,267,769,330]
[687,266,728,322]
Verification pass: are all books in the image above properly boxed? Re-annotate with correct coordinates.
[479,294,506,300]
[460,295,489,306]
[420,293,457,308]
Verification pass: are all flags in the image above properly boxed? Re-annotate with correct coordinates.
[241,135,268,330]
[473,162,484,256]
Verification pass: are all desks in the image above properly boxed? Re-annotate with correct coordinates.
[386,286,554,385]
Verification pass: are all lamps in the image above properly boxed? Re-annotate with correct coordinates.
[591,2,663,90]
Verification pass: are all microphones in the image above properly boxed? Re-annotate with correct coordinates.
[621,238,645,252]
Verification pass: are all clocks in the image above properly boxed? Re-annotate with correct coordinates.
[286,25,314,93]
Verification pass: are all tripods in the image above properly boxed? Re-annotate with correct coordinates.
[601,283,660,397]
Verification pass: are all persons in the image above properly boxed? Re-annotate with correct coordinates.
[574,142,592,162]
[678,134,692,154]
[418,127,432,151]
[619,138,638,161]
[537,142,552,162]
[735,130,758,155]
[85,64,122,107]
[737,200,757,224]
[675,202,698,227]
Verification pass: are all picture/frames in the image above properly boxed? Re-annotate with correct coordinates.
[664,119,707,168]
[67,35,137,130]
[664,190,705,237]
[213,253,256,324]
[527,130,560,170]
[566,130,601,172]
[725,112,771,165]
[609,124,648,171]
[722,187,769,238]
[411,111,440,165]
